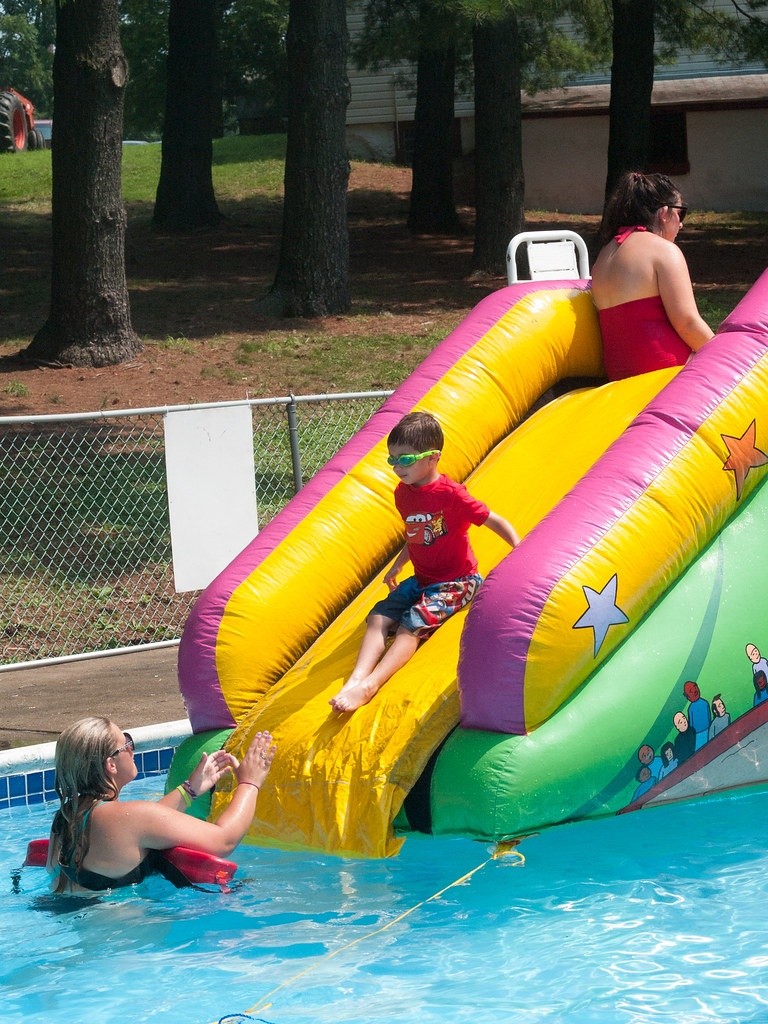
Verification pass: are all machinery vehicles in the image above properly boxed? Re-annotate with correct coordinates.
[0,87,45,156]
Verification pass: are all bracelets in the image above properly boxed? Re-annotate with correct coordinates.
[237,781,261,791]
[176,779,197,808]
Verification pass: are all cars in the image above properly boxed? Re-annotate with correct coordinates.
[34,120,53,149]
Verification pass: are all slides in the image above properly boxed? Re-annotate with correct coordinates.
[206,364,687,858]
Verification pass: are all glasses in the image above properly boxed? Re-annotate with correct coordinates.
[109,732,136,761]
[388,449,440,466]
[665,205,687,221]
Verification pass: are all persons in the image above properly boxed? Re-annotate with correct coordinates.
[326,411,525,719]
[590,172,716,383]
[30,715,278,928]
